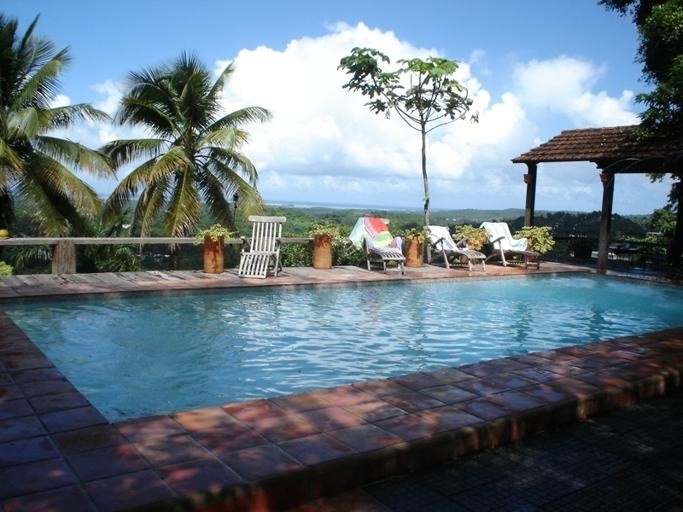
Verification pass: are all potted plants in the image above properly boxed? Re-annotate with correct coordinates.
[306,217,349,269]
[452,224,493,264]
[401,226,431,268]
[192,223,240,274]
[513,226,556,260]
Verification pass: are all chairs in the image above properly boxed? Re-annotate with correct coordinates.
[591,243,642,272]
[239,215,287,279]
[479,222,541,271]
[349,216,406,275]
[424,226,487,271]
